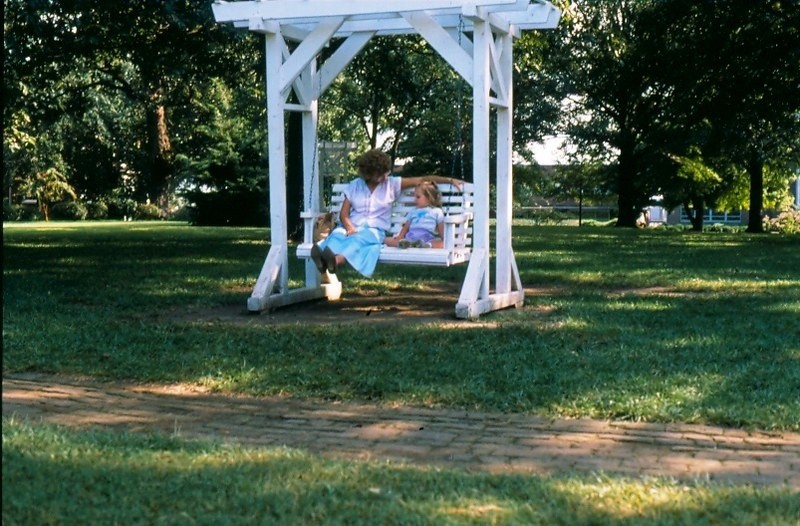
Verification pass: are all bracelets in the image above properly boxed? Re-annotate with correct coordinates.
[451,178,452,181]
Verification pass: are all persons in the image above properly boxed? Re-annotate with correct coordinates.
[310,149,463,278]
[384,179,444,248]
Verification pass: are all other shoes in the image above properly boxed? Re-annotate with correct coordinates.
[399,239,423,250]
[311,244,326,273]
[322,246,336,273]
